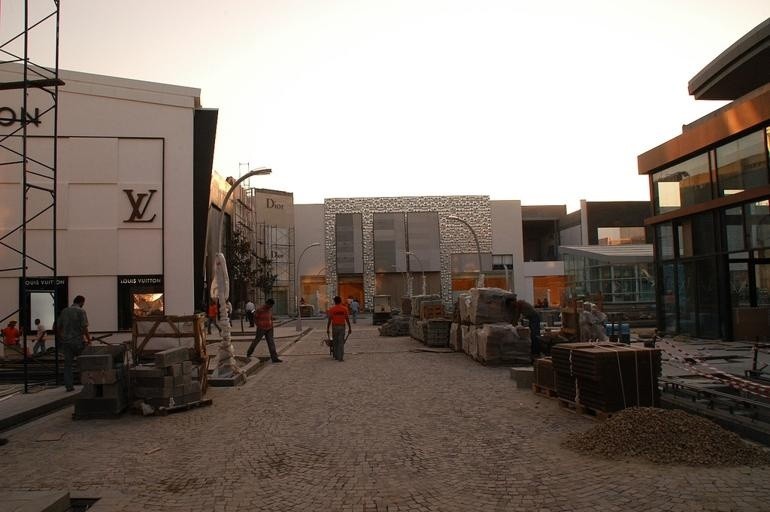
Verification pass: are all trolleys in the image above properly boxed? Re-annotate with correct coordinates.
[324,333,349,357]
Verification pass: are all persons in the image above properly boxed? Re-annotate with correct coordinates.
[350,298,360,324]
[31,319,48,354]
[0,320,24,345]
[57,296,91,392]
[505,297,540,355]
[207,300,232,335]
[246,299,283,363]
[327,296,351,361]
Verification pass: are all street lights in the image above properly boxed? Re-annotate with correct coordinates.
[215,169,272,375]
[392,265,405,296]
[296,242,321,331]
[446,216,484,287]
[406,252,426,295]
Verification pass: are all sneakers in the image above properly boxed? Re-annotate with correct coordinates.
[67,388,75,392]
[272,359,283,362]
